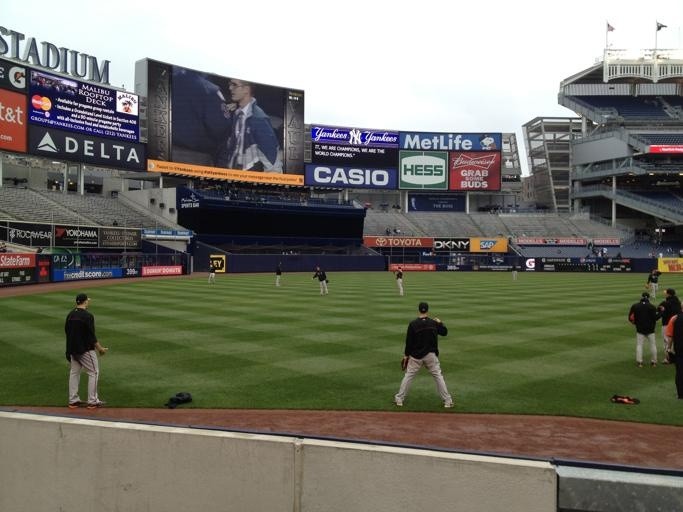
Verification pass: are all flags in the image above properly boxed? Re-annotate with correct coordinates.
[608,23,614,32]
[657,22,667,31]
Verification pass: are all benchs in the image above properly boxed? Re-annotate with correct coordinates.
[0,186,175,232]
[606,98,682,220]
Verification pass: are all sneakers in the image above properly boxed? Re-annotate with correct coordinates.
[638,358,669,368]
[87,404,104,409]
[444,403,454,408]
[395,394,403,406]
[69,400,87,409]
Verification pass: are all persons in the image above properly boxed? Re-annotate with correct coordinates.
[392,204,395,211]
[394,266,403,296]
[0,243,6,252]
[582,241,622,258]
[665,302,683,401]
[646,244,683,258]
[384,226,400,235]
[173,67,229,166]
[364,200,373,209]
[220,77,279,173]
[377,199,388,211]
[207,264,214,284]
[35,247,43,253]
[395,204,401,209]
[274,261,282,287]
[647,268,661,298]
[394,301,454,408]
[511,265,518,281]
[631,292,657,368]
[654,288,680,363]
[222,184,308,207]
[311,266,328,295]
[481,202,516,216]
[112,220,118,226]
[65,293,109,409]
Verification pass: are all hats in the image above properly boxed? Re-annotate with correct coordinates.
[419,303,429,311]
[76,294,91,303]
[664,289,675,296]
[642,293,650,298]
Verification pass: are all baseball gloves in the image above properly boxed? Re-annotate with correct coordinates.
[644,283,651,289]
[401,357,408,370]
